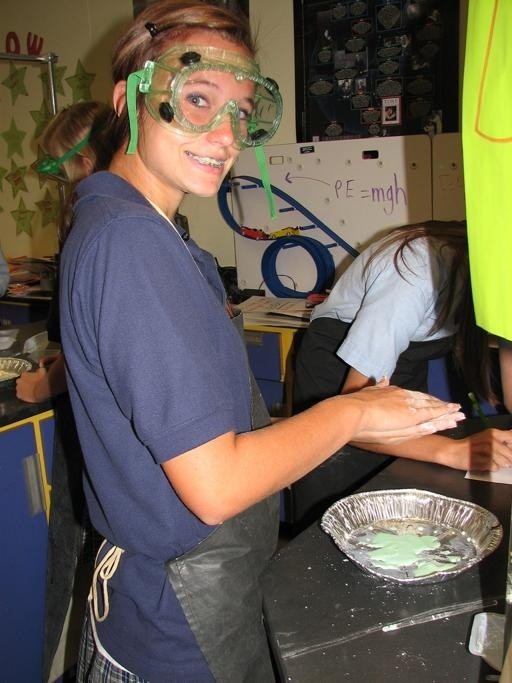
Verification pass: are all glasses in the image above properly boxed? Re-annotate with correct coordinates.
[139,44,284,149]
[30,132,98,184]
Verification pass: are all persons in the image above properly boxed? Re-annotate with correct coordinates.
[59,0,467,683]
[291,220,512,539]
[15,101,117,405]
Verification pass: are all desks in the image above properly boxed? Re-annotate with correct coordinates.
[260,413,510,679]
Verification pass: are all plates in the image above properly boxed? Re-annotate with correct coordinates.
[319,487,506,584]
[0,357,33,383]
